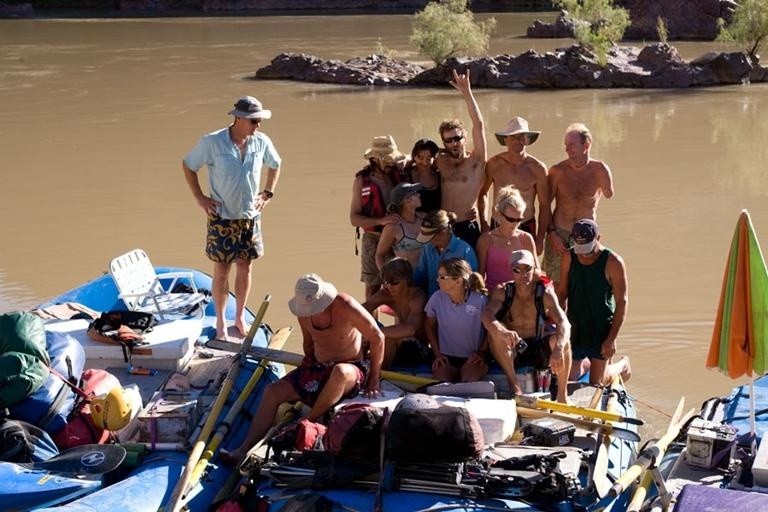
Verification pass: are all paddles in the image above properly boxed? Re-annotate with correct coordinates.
[164,293,296,512]
[586,375,696,512]
[206,339,644,443]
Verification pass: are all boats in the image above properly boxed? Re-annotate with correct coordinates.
[0,267,287,512]
[594,372,767,512]
[211,353,641,512]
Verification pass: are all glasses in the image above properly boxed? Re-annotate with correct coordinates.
[513,267,531,273]
[444,136,463,143]
[501,211,524,222]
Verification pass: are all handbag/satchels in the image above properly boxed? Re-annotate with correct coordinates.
[0,312,123,463]
[320,403,391,461]
[385,394,484,463]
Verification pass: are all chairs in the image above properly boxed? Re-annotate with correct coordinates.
[109,247,205,320]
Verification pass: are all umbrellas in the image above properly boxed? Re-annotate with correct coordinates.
[706,210,768,454]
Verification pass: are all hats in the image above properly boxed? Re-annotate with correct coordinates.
[227,96,271,119]
[288,275,337,317]
[416,209,449,244]
[389,183,421,204]
[509,250,535,268]
[572,219,599,254]
[495,117,540,145]
[365,136,405,162]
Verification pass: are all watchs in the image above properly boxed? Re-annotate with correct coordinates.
[263,190,274,198]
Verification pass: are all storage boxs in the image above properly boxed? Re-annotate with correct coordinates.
[519,415,576,447]
[685,416,738,474]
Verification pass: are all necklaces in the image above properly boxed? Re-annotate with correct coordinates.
[310,310,334,331]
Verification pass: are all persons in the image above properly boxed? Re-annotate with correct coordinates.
[182,95,282,342]
[350,67,631,406]
[218,272,385,466]
